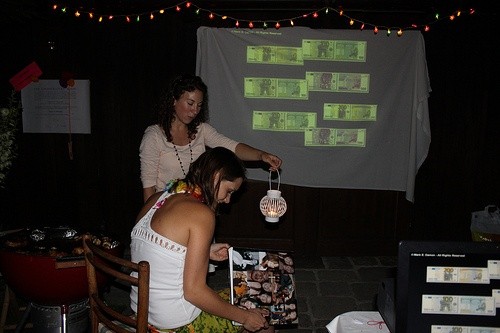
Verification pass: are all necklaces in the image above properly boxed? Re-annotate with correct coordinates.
[166,121,193,179]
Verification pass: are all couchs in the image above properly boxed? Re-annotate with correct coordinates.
[376,240,500,333]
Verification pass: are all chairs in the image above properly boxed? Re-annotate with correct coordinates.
[83,233,150,333]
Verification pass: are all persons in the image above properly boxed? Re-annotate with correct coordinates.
[128,146,272,333]
[140,74,282,204]
[226,247,300,330]
